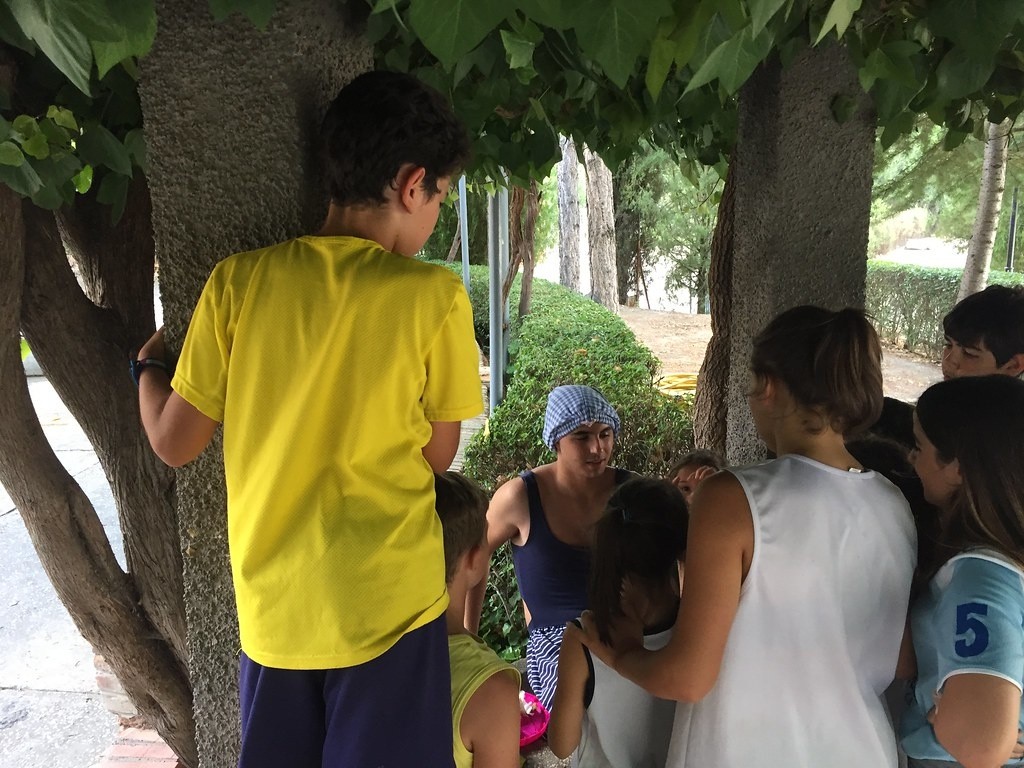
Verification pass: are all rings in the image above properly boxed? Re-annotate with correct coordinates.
[581,609,594,617]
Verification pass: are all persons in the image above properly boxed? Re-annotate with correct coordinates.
[431,471,522,768]
[547,477,690,768]
[141,70,485,768]
[465,385,649,719]
[567,284,1024,768]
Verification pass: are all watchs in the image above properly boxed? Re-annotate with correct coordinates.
[129,358,168,386]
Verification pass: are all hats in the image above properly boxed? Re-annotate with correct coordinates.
[543,384,620,454]
